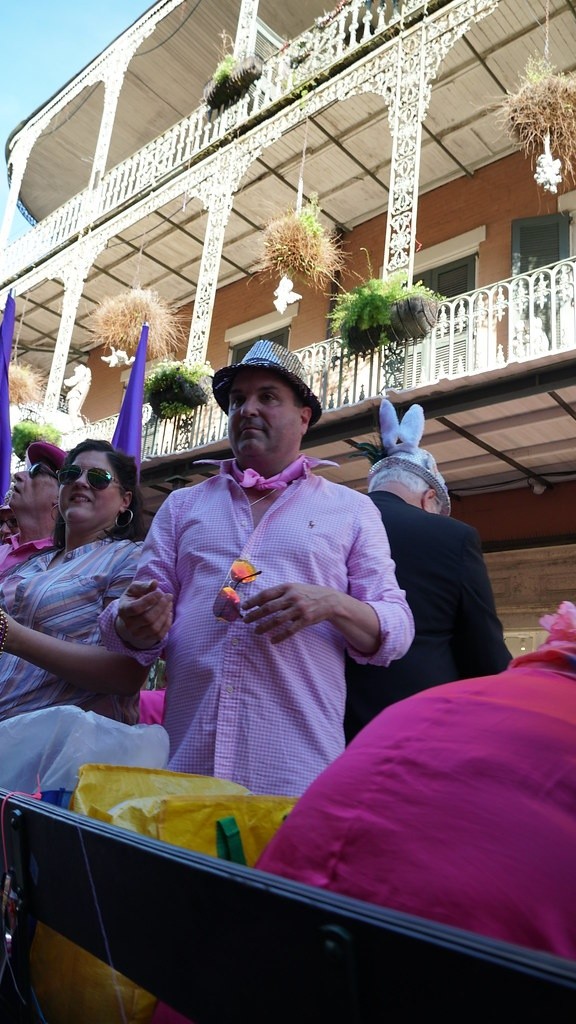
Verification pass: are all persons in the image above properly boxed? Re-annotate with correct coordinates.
[0,438,146,726]
[0,441,69,583]
[0,489,20,546]
[96,340,417,799]
[344,398,514,748]
[153,600,576,1023]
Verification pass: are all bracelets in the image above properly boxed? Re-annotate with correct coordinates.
[0,608,9,656]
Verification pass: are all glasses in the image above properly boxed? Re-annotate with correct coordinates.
[56,465,121,493]
[26,463,56,480]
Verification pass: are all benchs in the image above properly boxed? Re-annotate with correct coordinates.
[0,789,576,1023]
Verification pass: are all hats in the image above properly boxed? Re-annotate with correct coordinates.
[367,446,452,516]
[25,441,68,469]
[212,340,322,426]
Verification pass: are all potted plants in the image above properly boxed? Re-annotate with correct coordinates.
[204,53,265,109]
[324,248,447,356]
[144,357,215,419]
